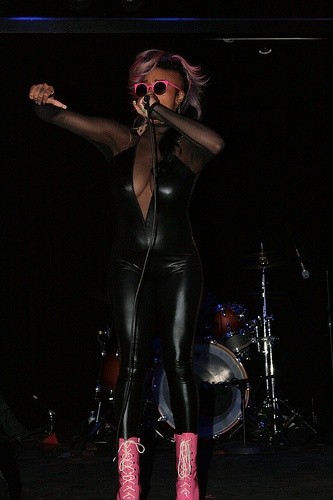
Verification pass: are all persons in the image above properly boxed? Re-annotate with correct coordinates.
[29,49,225,500]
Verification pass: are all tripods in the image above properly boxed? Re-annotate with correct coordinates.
[226,243,318,456]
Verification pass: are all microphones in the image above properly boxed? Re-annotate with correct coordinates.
[141,95,150,110]
[295,248,309,280]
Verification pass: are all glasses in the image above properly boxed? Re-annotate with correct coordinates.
[134,80,181,95]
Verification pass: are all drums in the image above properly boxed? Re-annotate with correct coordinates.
[196,301,251,342]
[150,340,249,438]
[219,333,256,361]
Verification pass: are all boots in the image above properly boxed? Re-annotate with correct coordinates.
[174,433,199,500]
[117,437,145,500]
